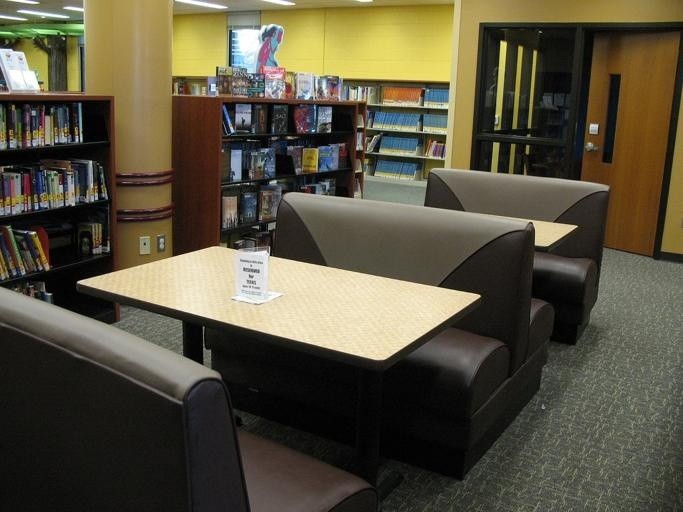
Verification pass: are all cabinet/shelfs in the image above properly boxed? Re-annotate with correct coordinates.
[173,94,359,258]
[354,100,367,199]
[338,76,450,187]
[0,88,122,324]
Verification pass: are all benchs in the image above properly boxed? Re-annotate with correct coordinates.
[421,167,610,345]
[0,284,378,510]
[204,191,555,481]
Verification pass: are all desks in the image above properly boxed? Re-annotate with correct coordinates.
[531,217,578,253]
[75,244,483,481]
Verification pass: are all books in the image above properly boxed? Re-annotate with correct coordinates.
[0,48,109,303]
